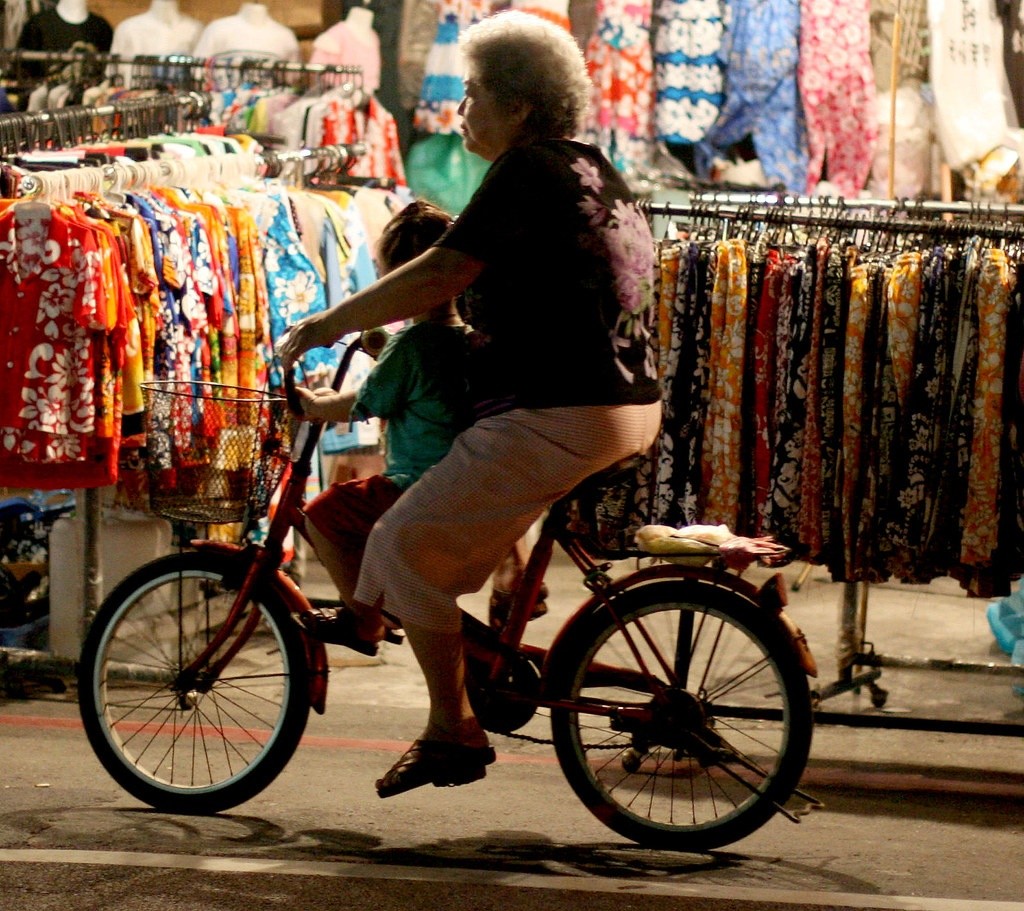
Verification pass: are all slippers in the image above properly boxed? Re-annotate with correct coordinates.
[377,739,497,799]
[489,583,547,627]
[291,599,405,658]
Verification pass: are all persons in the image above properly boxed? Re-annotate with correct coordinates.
[193,2,299,62]
[306,6,383,95]
[278,9,665,801]
[110,0,202,59]
[297,197,469,658]
[17,0,113,53]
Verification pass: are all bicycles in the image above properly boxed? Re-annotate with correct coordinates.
[78,329,828,854]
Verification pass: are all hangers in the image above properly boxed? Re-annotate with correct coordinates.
[0,46,370,177]
[14,142,397,211]
[632,191,1023,266]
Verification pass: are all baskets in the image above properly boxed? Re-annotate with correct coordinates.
[140,380,289,523]
